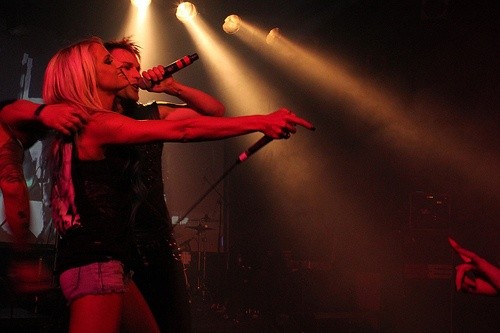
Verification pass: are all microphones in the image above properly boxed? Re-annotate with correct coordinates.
[235,126,289,165]
[138,52,199,90]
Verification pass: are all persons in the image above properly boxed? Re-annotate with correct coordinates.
[449,237,499,294]
[1,34,226,332]
[42,36,315,332]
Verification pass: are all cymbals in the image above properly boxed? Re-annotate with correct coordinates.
[186,225,216,230]
[191,217,218,222]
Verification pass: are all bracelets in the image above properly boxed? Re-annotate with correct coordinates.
[34,103,48,119]
